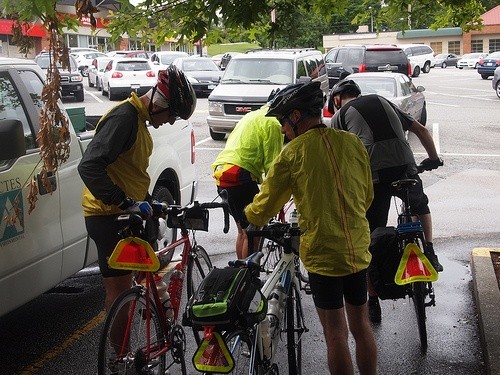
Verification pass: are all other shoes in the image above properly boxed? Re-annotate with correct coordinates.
[109,353,159,375]
[367,296,382,321]
[424,246,444,272]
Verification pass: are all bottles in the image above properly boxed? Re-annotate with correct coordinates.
[260,317,273,357]
[166,262,183,309]
[153,273,175,317]
[266,287,284,339]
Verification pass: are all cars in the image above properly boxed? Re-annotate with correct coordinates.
[477,51,500,79]
[434,53,462,68]
[221,52,242,68]
[321,72,427,142]
[457,53,488,69]
[492,66,500,98]
[210,54,225,66]
[149,51,188,75]
[167,57,223,96]
[408,60,421,77]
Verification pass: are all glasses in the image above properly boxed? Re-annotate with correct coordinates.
[276,107,296,125]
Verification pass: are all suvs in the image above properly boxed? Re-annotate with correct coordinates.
[323,44,411,89]
[70,48,154,92]
[34,55,84,101]
[100,59,157,100]
[395,44,436,74]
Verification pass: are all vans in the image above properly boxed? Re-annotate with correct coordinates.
[207,48,329,141]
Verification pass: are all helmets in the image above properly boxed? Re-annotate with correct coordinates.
[155,64,196,121]
[328,80,361,116]
[265,81,324,121]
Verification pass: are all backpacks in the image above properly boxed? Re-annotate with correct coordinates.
[185,264,269,327]
[367,226,407,300]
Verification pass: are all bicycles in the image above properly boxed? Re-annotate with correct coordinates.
[180,197,312,375]
[392,159,444,351]
[97,200,230,375]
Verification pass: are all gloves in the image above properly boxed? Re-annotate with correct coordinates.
[150,200,168,220]
[126,201,153,220]
[420,158,442,171]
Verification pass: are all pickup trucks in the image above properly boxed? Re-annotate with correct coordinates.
[0,55,109,317]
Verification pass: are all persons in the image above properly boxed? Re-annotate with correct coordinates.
[327,80,443,315]
[77,64,197,364]
[211,88,285,258]
[240,81,379,375]
